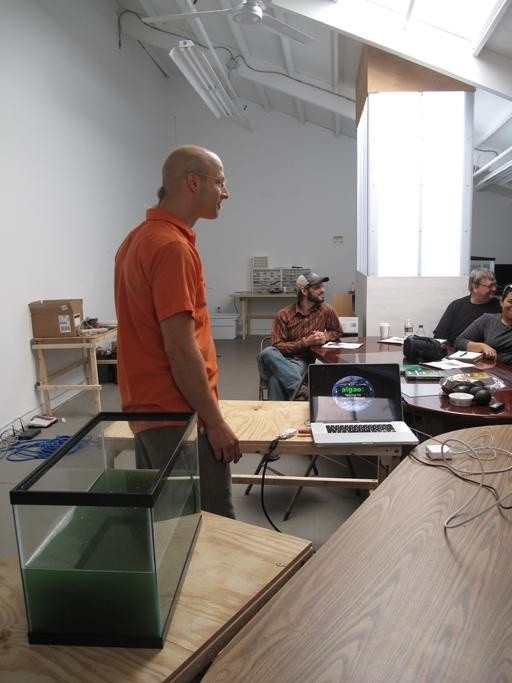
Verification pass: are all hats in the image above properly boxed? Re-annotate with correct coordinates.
[296,271,330,289]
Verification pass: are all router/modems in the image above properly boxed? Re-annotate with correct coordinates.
[12,421,41,439]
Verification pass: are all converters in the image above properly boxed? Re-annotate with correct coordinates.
[424,444,453,459]
[283,427,297,437]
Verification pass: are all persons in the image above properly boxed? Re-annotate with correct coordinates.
[115,144,242,520]
[256,271,344,461]
[454,285,512,368]
[432,267,502,347]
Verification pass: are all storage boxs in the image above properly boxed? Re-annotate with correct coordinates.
[333,294,359,334]
[28,299,83,337]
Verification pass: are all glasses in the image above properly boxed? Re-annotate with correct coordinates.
[477,282,498,289]
[189,172,224,185]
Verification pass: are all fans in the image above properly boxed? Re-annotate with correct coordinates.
[141,1,315,45]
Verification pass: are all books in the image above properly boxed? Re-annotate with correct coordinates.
[448,350,484,362]
[399,364,423,374]
[404,370,447,381]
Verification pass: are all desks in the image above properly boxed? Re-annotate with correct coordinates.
[309,337,512,441]
[233,291,298,341]
[30,321,117,416]
[200,424,512,683]
[100,400,404,498]
[0,510,313,683]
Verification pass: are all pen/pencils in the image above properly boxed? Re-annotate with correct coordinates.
[328,343,342,346]
[460,351,468,357]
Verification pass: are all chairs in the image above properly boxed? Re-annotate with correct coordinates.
[245,372,362,521]
[259,336,278,400]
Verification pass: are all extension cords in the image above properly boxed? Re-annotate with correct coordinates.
[79,327,108,333]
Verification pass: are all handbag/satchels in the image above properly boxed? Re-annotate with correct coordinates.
[403,334,448,363]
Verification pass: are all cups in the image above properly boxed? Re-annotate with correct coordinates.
[379,323,390,339]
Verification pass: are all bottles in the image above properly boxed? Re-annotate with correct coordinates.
[417,324,425,337]
[404,319,413,336]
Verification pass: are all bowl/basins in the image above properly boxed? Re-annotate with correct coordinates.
[448,392,474,408]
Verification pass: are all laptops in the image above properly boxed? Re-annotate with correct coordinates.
[308,362,420,447]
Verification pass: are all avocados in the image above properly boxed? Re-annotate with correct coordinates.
[469,386,485,395]
[453,385,469,393]
[474,390,492,406]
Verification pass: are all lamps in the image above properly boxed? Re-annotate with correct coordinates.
[168,40,245,119]
